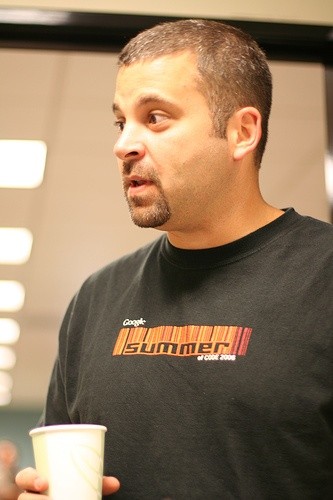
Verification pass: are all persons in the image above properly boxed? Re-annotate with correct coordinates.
[16,19,333,500]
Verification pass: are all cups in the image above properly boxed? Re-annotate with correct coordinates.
[29,424,107,500]
